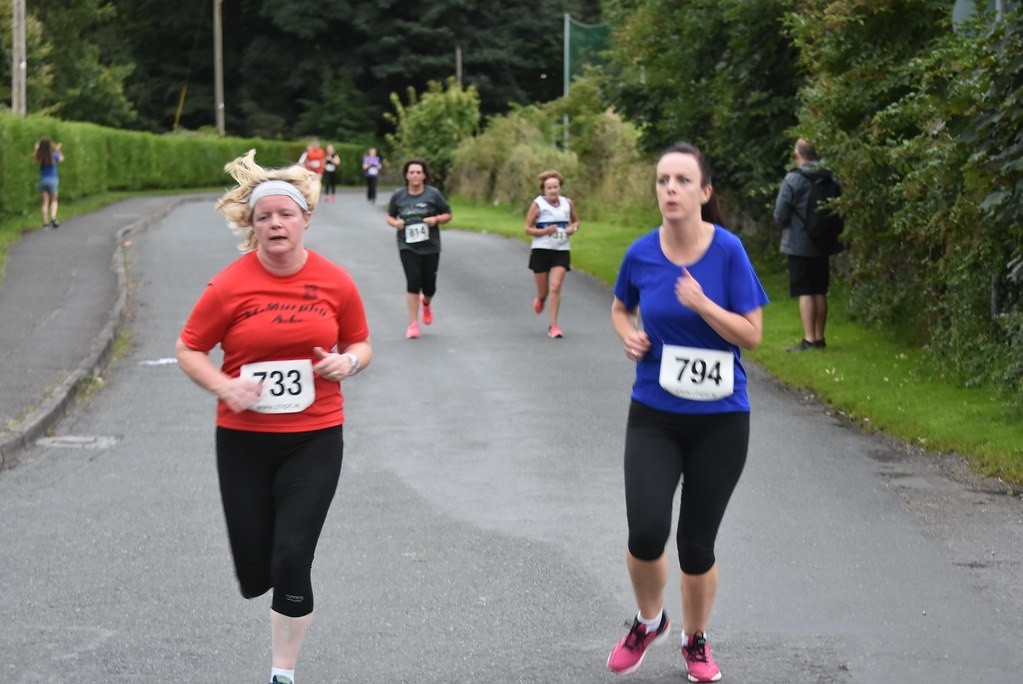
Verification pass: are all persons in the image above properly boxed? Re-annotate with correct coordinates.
[386,159,453,338]
[363,148,382,204]
[298,141,340,202]
[606,141,770,683]
[175,147,371,684]
[525,170,581,338]
[773,139,846,352]
[32,138,64,230]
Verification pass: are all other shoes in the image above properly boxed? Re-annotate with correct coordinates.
[43,224,50,228]
[406,321,419,338]
[533,297,544,313]
[547,325,563,338]
[420,294,432,325]
[50,218,58,228]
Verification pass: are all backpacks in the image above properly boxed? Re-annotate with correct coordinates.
[789,167,846,255]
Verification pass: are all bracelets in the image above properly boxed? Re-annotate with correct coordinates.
[344,354,360,375]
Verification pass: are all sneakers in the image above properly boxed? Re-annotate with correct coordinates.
[786,338,817,352]
[606,607,671,676]
[681,629,722,682]
[816,338,826,347]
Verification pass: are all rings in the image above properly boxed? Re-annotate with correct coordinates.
[634,353,637,357]
